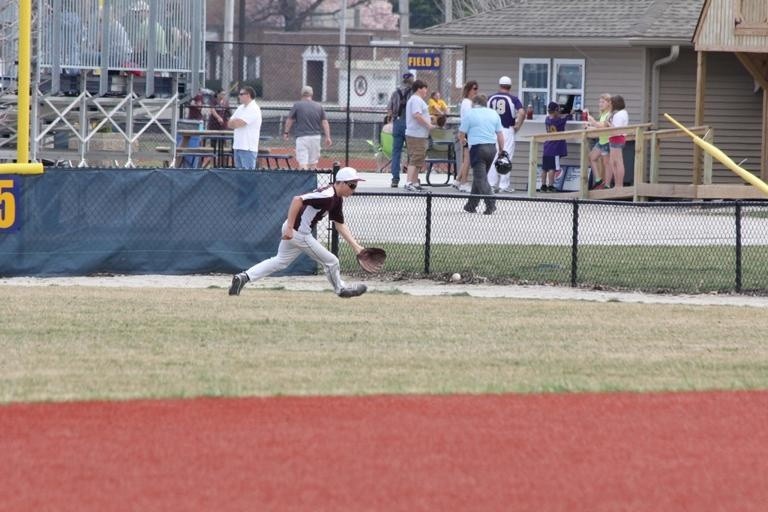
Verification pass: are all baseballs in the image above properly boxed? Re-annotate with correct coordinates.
[452,273,461,281]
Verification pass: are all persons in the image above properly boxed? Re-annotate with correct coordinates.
[44,0,190,77]
[457,93,505,215]
[283,86,332,170]
[452,80,479,193]
[540,102,572,193]
[587,94,613,188]
[227,86,262,169]
[606,95,629,188]
[487,76,525,193]
[207,88,232,147]
[188,89,204,119]
[382,73,449,193]
[228,167,366,298]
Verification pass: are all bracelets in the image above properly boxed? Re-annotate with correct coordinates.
[284,131,288,134]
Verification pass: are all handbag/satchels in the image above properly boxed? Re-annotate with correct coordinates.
[494,151,511,175]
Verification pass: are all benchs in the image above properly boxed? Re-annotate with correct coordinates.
[157,146,294,170]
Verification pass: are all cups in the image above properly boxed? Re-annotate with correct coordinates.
[572,112,587,121]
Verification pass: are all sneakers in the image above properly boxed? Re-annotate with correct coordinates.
[413,184,428,190]
[452,179,515,215]
[592,176,609,190]
[404,181,422,193]
[540,184,548,193]
[546,186,557,193]
[229,273,249,296]
[338,284,367,298]
[391,179,398,187]
[403,167,408,173]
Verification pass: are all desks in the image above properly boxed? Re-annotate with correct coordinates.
[176,130,272,141]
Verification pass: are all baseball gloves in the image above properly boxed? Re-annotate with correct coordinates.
[357,247,386,274]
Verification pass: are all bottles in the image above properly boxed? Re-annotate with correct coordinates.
[527,104,533,119]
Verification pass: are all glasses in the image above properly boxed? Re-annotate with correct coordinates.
[473,87,477,91]
[239,93,248,96]
[344,181,356,189]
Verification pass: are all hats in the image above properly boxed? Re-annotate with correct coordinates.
[548,102,559,111]
[301,85,314,97]
[335,167,366,181]
[498,76,512,85]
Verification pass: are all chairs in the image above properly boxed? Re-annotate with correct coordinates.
[379,129,459,186]
